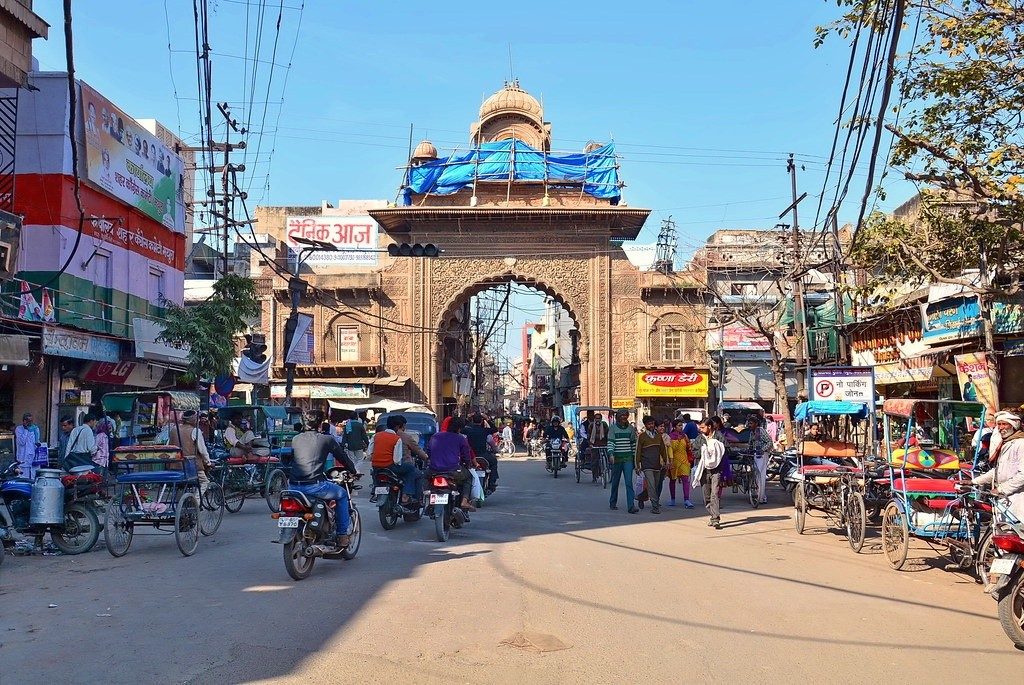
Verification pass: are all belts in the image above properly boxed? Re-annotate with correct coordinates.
[290,477,323,486]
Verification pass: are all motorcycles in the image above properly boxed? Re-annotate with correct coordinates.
[544,435,570,479]
[429,461,476,542]
[269,466,365,581]
[368,455,432,530]
[0,460,105,555]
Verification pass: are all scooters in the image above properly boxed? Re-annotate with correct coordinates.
[765,446,993,523]
[983,535,1024,650]
[471,456,497,508]
[526,437,543,457]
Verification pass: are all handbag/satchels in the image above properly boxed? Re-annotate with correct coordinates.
[468,468,481,500]
[32,446,48,467]
[634,472,650,500]
[62,452,94,475]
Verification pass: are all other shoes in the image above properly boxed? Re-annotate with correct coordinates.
[628,507,639,513]
[668,499,675,506]
[487,481,496,488]
[705,501,711,508]
[560,462,567,467]
[545,464,552,469]
[759,497,768,504]
[638,500,644,509]
[652,503,660,514]
[610,501,618,510]
[684,500,694,508]
[708,520,720,528]
[338,534,352,546]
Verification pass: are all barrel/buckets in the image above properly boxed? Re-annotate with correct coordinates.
[29,469,66,523]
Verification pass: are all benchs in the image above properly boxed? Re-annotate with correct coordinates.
[109,445,199,485]
[797,441,866,476]
[223,451,281,463]
[920,497,992,513]
[890,446,964,495]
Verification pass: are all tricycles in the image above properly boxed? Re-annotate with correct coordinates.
[200,405,305,513]
[793,400,871,553]
[881,398,1024,602]
[716,401,765,509]
[374,412,436,451]
[101,390,225,557]
[571,405,614,490]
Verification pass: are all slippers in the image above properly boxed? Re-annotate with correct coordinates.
[400,495,413,505]
[462,503,476,512]
[370,495,378,503]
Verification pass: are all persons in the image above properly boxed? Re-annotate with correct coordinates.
[693,417,727,529]
[166,409,215,511]
[288,409,361,548]
[607,410,637,514]
[424,416,477,513]
[64,413,98,475]
[15,412,42,480]
[223,410,279,464]
[748,417,773,505]
[543,416,570,468]
[958,411,1024,529]
[15,409,1024,548]
[500,419,514,458]
[635,416,667,514]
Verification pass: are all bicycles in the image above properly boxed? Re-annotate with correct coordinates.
[496,438,516,459]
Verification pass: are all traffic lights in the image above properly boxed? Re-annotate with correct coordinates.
[284,318,298,369]
[724,357,732,384]
[710,354,721,390]
[387,243,439,257]
[242,334,267,364]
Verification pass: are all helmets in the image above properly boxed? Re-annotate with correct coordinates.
[551,415,561,424]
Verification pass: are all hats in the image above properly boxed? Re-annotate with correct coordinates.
[994,411,1021,430]
